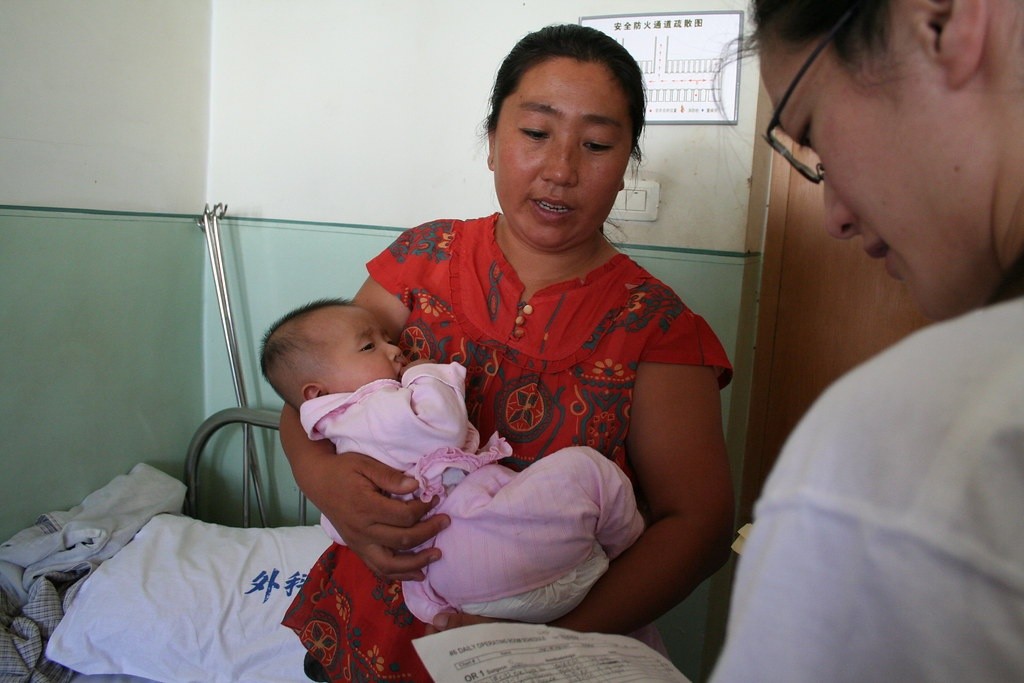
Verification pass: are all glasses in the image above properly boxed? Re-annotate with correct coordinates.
[763,10,853,187]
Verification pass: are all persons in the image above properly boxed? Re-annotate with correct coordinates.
[280,24,735,683]
[258,298,645,626]
[706,0,1024,683]
